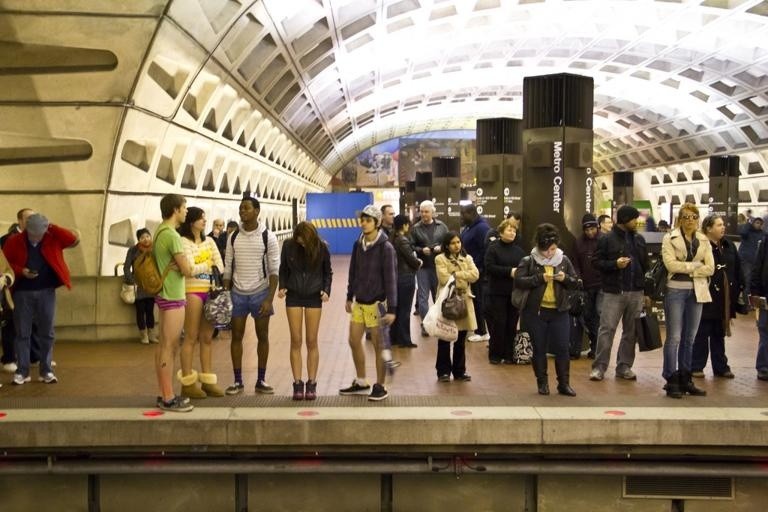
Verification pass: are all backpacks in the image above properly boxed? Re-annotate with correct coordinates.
[134,228,174,296]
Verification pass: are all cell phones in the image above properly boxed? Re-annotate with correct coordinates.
[550,273,562,278]
[29,270,38,274]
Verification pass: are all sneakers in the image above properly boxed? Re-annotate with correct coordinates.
[532,361,767,398]
[3,360,57,384]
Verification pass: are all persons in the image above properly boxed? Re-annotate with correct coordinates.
[175,206,225,398]
[747,229,768,379]
[0,249,15,358]
[690,214,744,379]
[378,199,767,365]
[433,231,480,383]
[2,214,81,384]
[281,220,333,400]
[657,202,716,399]
[150,193,195,411]
[340,203,399,401]
[515,222,580,396]
[589,205,650,381]
[225,197,282,396]
[120,216,239,347]
[0,206,57,374]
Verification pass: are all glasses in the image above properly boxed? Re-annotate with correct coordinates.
[683,214,698,219]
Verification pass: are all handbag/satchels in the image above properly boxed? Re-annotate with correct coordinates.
[120,283,137,304]
[442,257,667,364]
[205,288,234,325]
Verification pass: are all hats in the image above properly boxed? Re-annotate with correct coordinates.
[27,214,48,234]
[356,205,382,226]
[617,206,638,224]
[583,215,597,228]
